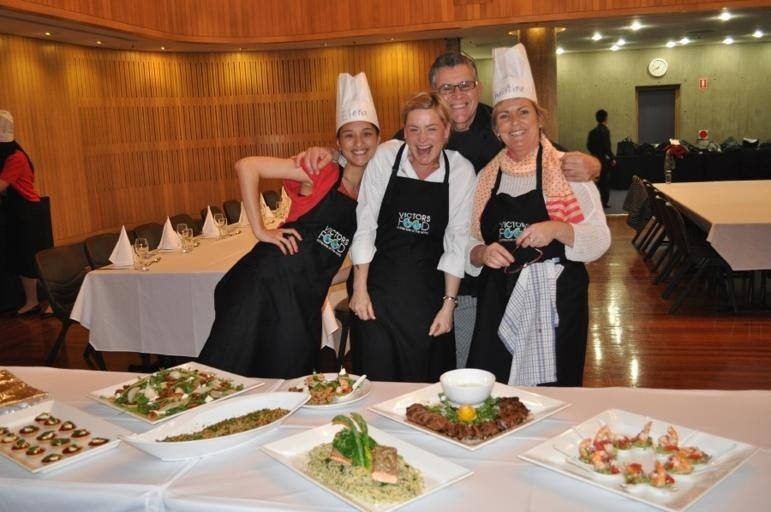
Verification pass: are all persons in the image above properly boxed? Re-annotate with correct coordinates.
[347,92,476,383]
[197,71,381,379]
[0,109,54,319]
[588,110,617,208]
[465,45,611,387]
[288,53,610,188]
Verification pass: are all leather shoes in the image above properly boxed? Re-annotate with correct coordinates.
[40,312,54,319]
[10,304,41,316]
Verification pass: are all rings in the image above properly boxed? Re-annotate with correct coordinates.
[528,237,533,242]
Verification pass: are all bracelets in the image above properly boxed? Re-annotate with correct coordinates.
[443,296,458,305]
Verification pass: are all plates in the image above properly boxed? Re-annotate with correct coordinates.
[87,360,264,426]
[518,407,763,511]
[256,420,475,512]
[367,380,572,451]
[117,392,313,462]
[0,399,122,473]
[282,373,373,408]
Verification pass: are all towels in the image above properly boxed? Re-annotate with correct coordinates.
[497,256,565,387]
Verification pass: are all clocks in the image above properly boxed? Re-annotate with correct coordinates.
[648,58,668,77]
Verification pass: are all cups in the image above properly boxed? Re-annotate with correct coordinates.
[186,228,196,250]
[275,201,283,218]
[666,170,671,184]
[213,213,224,239]
[134,237,149,271]
[176,223,188,253]
[219,219,230,237]
[131,245,139,269]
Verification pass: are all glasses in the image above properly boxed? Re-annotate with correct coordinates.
[504,244,543,275]
[438,80,477,95]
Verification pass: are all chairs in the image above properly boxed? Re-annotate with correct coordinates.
[262,190,279,210]
[662,201,740,316]
[34,242,151,372]
[642,184,662,251]
[651,195,728,300]
[223,200,241,224]
[201,206,221,224]
[133,223,163,250]
[632,178,657,249]
[169,214,197,237]
[643,189,673,271]
[84,230,135,271]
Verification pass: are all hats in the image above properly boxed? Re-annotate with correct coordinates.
[335,72,381,135]
[491,44,537,107]
[0,110,17,143]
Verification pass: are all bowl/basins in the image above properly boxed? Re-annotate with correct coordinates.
[440,368,496,408]
